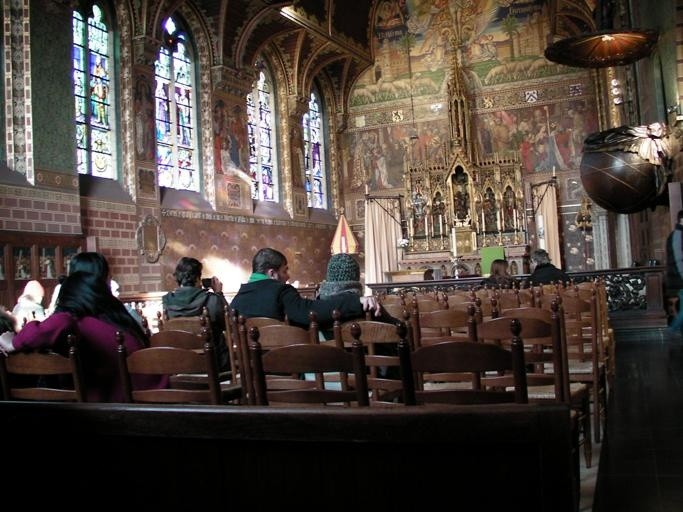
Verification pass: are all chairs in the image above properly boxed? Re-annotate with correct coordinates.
[376,278,607,313]
[466,301,580,505]
[339,310,418,408]
[161,304,240,388]
[231,308,325,405]
[529,289,608,444]
[0,332,84,403]
[249,323,370,407]
[224,305,299,385]
[397,318,527,405]
[238,310,351,408]
[141,317,242,405]
[400,292,483,352]
[465,297,594,469]
[115,328,223,405]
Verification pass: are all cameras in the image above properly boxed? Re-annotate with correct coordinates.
[202,278,215,288]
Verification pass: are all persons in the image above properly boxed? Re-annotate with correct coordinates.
[479,259,519,290]
[521,250,572,288]
[226,248,380,380]
[12,280,46,335]
[0,252,170,403]
[163,257,232,374]
[310,253,421,379]
[662,209,683,329]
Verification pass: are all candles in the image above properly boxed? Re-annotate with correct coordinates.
[513,208,517,229]
[424,214,429,235]
[410,217,414,237]
[439,214,443,234]
[481,211,486,231]
[452,228,457,257]
[553,165,556,178]
[496,211,501,231]
[365,184,369,194]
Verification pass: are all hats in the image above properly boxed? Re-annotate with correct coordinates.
[324,251,362,286]
